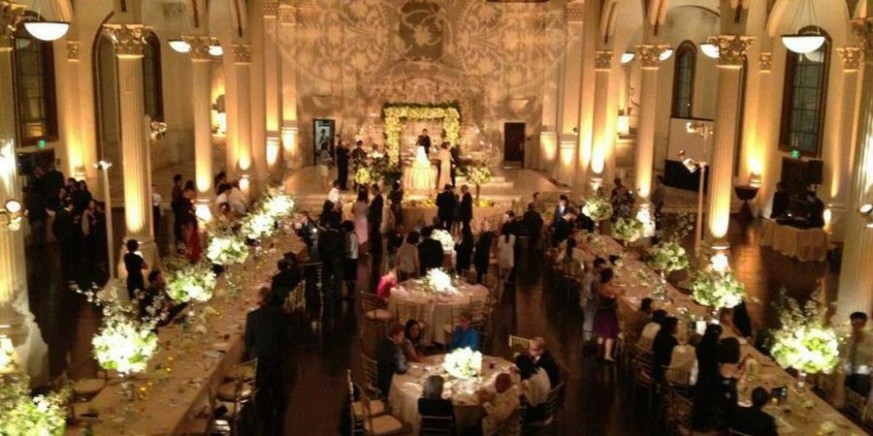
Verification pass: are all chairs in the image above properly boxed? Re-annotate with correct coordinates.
[191,238,731,436]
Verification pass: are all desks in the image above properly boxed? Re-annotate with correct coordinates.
[405,166,438,190]
[756,217,828,262]
[401,203,438,230]
[390,350,519,435]
[388,280,490,343]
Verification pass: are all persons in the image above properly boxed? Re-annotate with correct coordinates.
[771,182,788,217]
[151,183,165,238]
[837,312,873,409]
[216,167,260,229]
[246,128,778,436]
[172,173,203,265]
[803,191,825,228]
[22,160,111,307]
[125,239,172,320]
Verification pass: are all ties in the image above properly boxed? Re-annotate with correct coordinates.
[850,338,859,374]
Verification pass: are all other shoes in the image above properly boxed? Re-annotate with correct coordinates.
[325,301,332,309]
[604,358,618,364]
[343,294,353,301]
[363,252,370,257]
[336,302,343,309]
[595,356,603,362]
[582,346,596,353]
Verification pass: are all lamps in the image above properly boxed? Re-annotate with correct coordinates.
[700,8,720,57]
[684,122,713,259]
[23,0,69,42]
[780,0,826,52]
[168,12,192,52]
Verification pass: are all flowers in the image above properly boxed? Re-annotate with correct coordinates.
[687,250,742,310]
[0,365,70,436]
[581,197,615,222]
[766,290,842,379]
[206,234,249,265]
[643,237,688,275]
[264,190,296,216]
[612,217,645,245]
[89,308,160,374]
[238,210,275,239]
[161,260,219,303]
[591,235,872,436]
